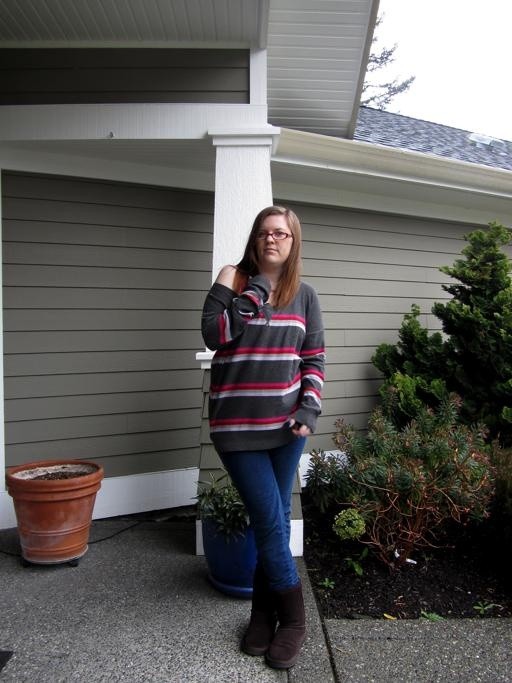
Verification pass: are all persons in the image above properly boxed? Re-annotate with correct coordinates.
[199,204,328,670]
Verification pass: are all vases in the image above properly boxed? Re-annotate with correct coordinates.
[6,457,108,563]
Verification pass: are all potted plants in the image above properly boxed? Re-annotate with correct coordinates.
[154,464,258,599]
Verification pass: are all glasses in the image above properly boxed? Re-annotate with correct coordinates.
[257,232,295,241]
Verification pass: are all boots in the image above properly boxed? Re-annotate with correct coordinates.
[267,581,306,668]
[241,567,276,656]
